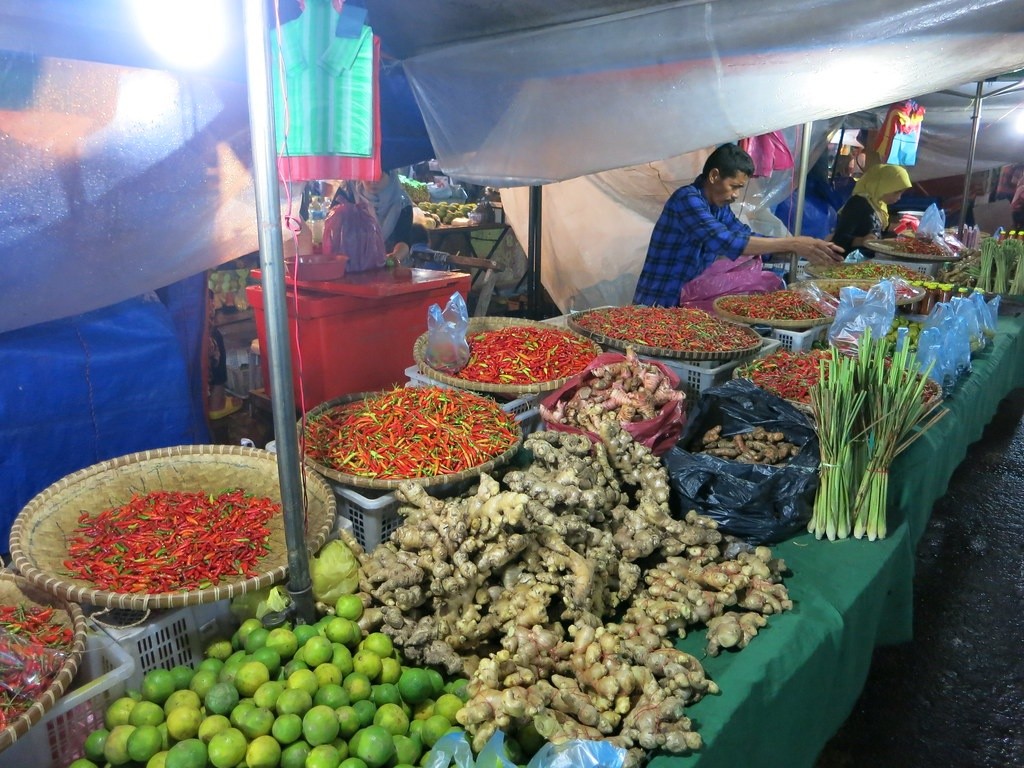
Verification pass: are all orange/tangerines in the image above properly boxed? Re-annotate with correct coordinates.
[67,594,548,768]
[883,315,926,349]
[419,200,473,225]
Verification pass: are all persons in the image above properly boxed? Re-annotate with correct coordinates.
[828,165,912,263]
[328,167,412,267]
[630,142,845,310]
[850,147,865,178]
[995,161,1024,230]
[207,289,243,421]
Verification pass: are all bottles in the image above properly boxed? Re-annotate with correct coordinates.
[308,196,331,244]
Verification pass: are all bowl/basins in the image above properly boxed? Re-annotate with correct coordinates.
[284,254,349,281]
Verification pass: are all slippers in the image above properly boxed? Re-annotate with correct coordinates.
[208,394,243,420]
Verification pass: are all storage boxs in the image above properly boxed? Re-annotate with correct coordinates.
[246,264,472,410]
[734,323,831,355]
[605,337,784,416]
[264,440,431,552]
[97,598,232,677]
[405,382,545,437]
[773,260,811,280]
[0,621,137,768]
[872,255,942,276]
[404,364,549,416]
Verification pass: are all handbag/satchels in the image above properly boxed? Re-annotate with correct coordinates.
[426,290,470,374]
[324,179,388,273]
[425,730,629,768]
[914,290,1000,400]
[827,279,897,356]
[914,201,946,241]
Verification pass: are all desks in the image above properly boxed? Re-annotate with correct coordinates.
[428,223,513,297]
[643,308,1024,768]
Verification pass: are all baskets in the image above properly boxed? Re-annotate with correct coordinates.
[284,252,348,280]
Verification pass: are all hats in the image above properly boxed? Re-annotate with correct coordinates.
[827,128,866,149]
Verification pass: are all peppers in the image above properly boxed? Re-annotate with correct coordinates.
[300,385,522,479]
[716,290,840,320]
[62,488,279,594]
[575,304,758,352]
[810,262,929,304]
[0,603,73,736]
[738,348,937,407]
[426,324,598,386]
[890,231,954,257]
